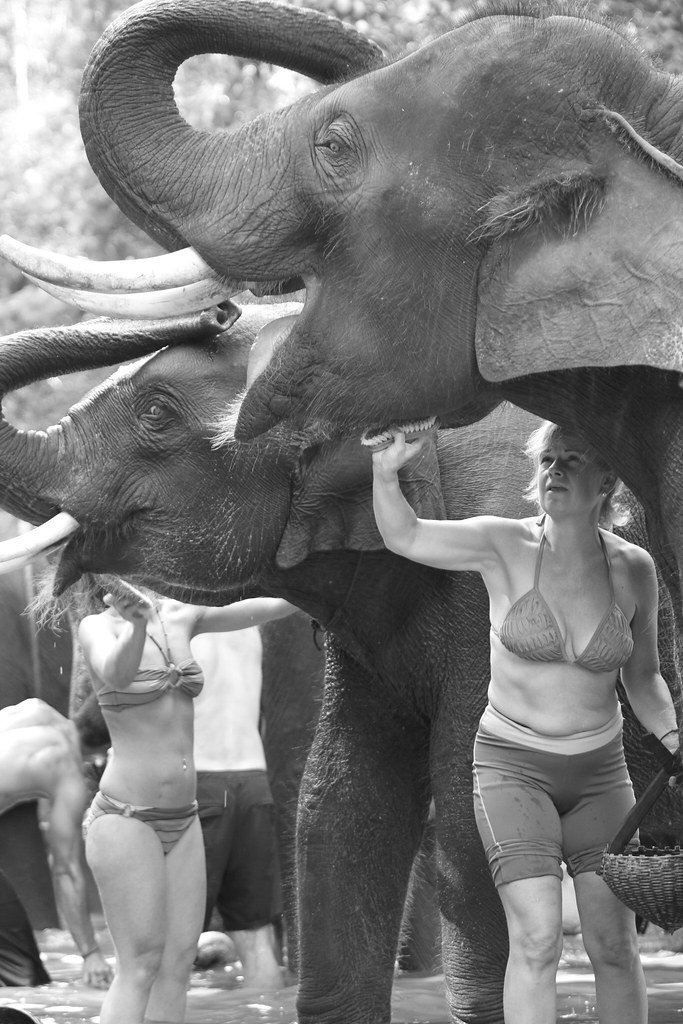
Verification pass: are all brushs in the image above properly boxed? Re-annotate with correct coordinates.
[94,572,154,611]
[360,415,441,452]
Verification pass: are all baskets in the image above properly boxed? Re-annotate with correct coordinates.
[601,842,683,931]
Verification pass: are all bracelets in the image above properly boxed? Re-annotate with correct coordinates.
[82,947,98,958]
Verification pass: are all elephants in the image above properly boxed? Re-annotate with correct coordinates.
[2,2,683,1024]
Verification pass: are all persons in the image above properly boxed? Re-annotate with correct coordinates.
[190,625,282,989]
[0,689,117,988]
[76,572,302,1024]
[370,418,683,1024]
